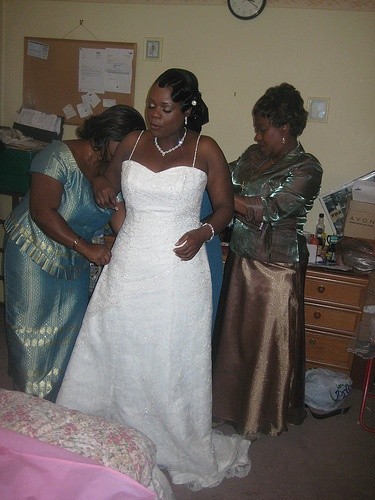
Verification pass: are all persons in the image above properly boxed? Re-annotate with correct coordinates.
[199,189,224,335]
[56,68,252,489]
[211,81,324,443]
[2,104,146,404]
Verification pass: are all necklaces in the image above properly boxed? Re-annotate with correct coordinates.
[76,153,100,178]
[270,141,296,165]
[154,126,187,156]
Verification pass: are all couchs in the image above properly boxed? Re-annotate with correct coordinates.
[1,387,173,500]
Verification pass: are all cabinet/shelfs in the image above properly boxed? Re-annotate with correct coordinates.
[304,249,371,379]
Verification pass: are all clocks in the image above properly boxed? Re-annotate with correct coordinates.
[227,0,267,20]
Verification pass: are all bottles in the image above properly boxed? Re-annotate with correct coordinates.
[316,214,325,256]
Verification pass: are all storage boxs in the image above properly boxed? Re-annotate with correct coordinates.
[341,198,375,241]
[0,194,25,252]
[0,125,44,194]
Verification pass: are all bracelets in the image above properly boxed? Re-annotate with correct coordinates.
[73,236,82,250]
[202,223,214,242]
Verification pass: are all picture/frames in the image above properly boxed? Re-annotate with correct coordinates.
[306,97,329,123]
[143,37,162,62]
